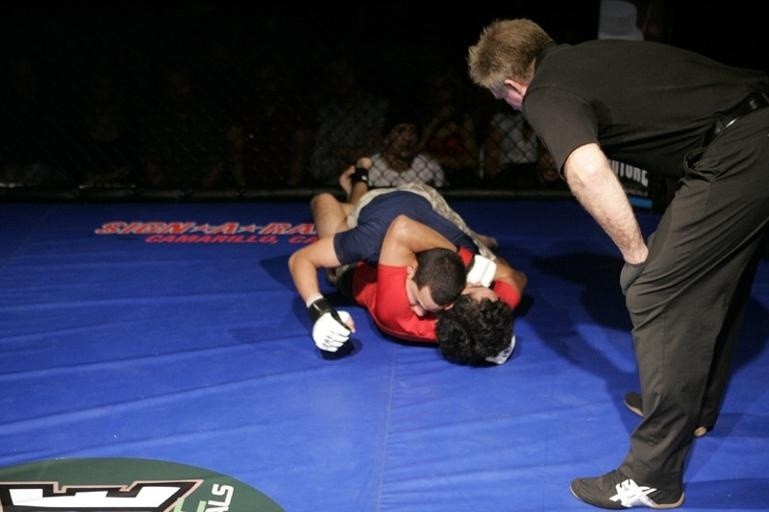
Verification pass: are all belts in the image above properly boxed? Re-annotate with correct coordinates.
[703,91,769,150]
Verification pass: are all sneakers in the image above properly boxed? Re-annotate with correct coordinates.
[624,391,708,438]
[568,467,685,510]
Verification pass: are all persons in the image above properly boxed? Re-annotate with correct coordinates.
[308,188,524,363]
[152,101,569,194]
[289,182,522,354]
[469,19,769,509]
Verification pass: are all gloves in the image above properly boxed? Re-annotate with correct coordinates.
[484,333,516,365]
[305,292,356,353]
